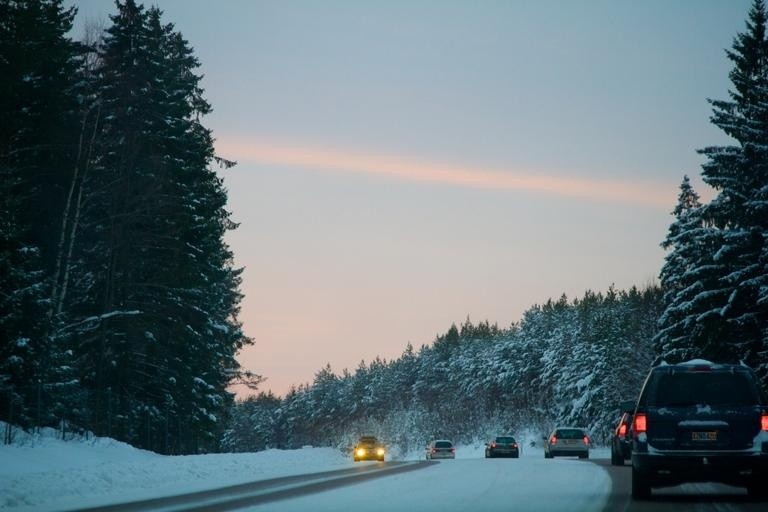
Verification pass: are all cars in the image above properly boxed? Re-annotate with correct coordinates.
[628,360,767,501]
[352,435,385,461]
[424,438,456,460]
[484,436,519,457]
[609,413,633,467]
[541,426,590,458]
